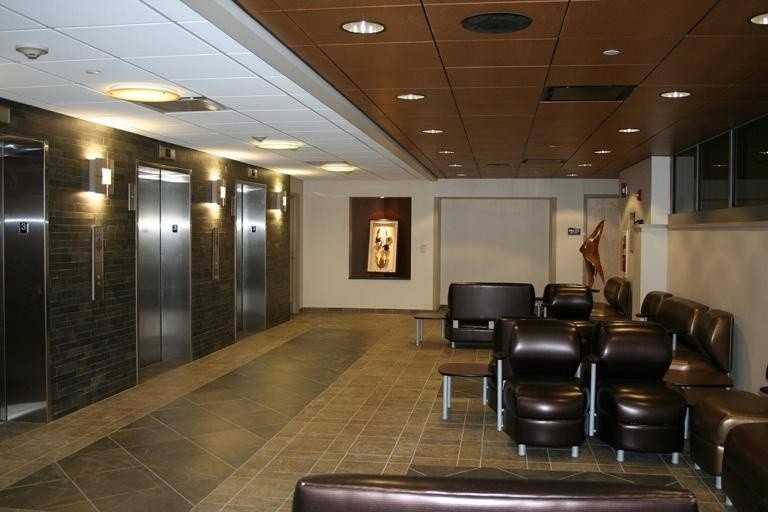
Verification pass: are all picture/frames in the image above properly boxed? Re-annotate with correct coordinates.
[367,219,399,274]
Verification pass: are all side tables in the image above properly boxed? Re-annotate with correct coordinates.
[438,362,495,420]
[414,312,445,345]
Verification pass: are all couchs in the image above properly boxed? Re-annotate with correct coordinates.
[290,474,696,511]
